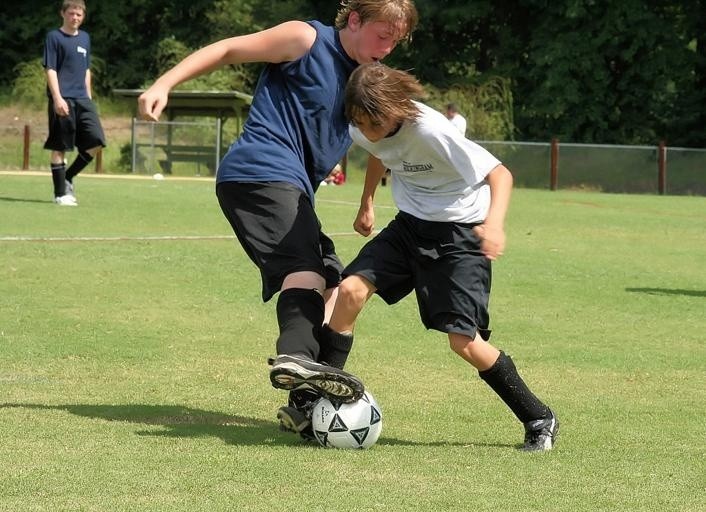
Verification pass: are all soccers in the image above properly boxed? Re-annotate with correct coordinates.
[312,391,382,449]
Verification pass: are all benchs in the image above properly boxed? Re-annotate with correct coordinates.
[156,143,230,177]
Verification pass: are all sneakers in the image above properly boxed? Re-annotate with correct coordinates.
[523,405,559,451]
[54,179,76,206]
[278,405,318,441]
[267,355,363,403]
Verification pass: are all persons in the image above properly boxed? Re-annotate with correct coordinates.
[276,62,558,453]
[446,104,467,138]
[136,0,421,432]
[42,0,106,206]
